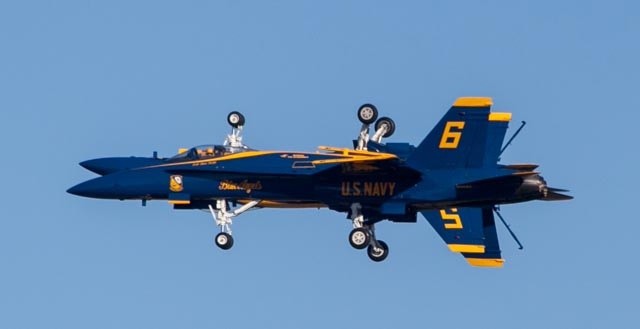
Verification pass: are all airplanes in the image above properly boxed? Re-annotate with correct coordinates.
[66,96,574,256]
[78,110,573,270]
